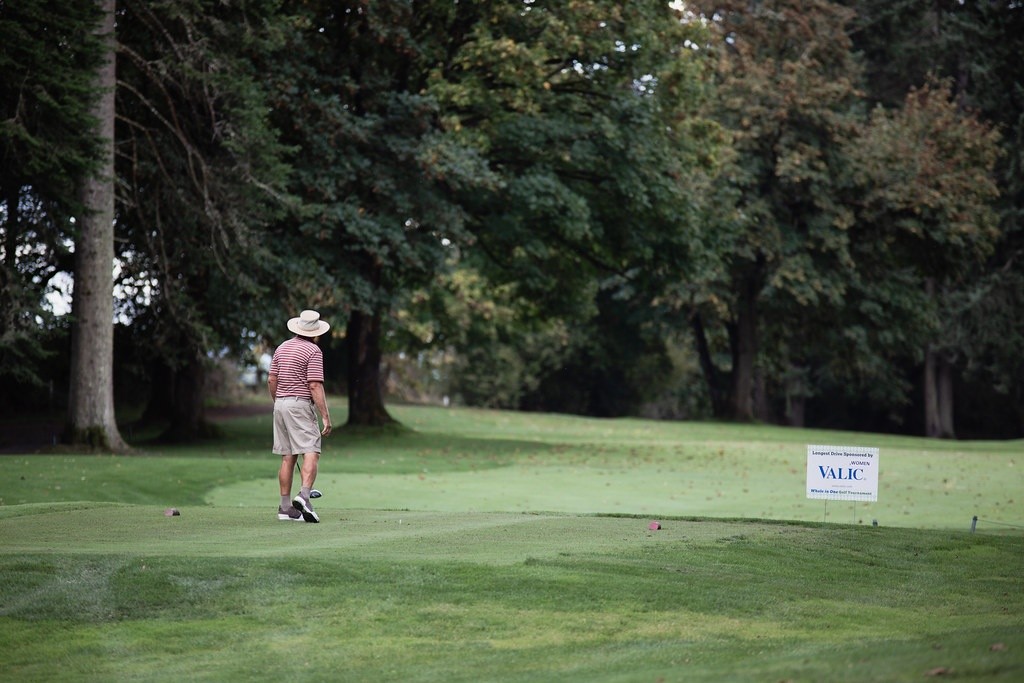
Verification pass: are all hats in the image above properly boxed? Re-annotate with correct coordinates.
[287,310,330,337]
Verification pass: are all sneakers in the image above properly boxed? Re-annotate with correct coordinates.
[293,493,320,522]
[278,505,304,521]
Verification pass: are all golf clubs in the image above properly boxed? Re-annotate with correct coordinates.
[296,461,323,498]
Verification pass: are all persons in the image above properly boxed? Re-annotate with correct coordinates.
[269,310,331,523]
